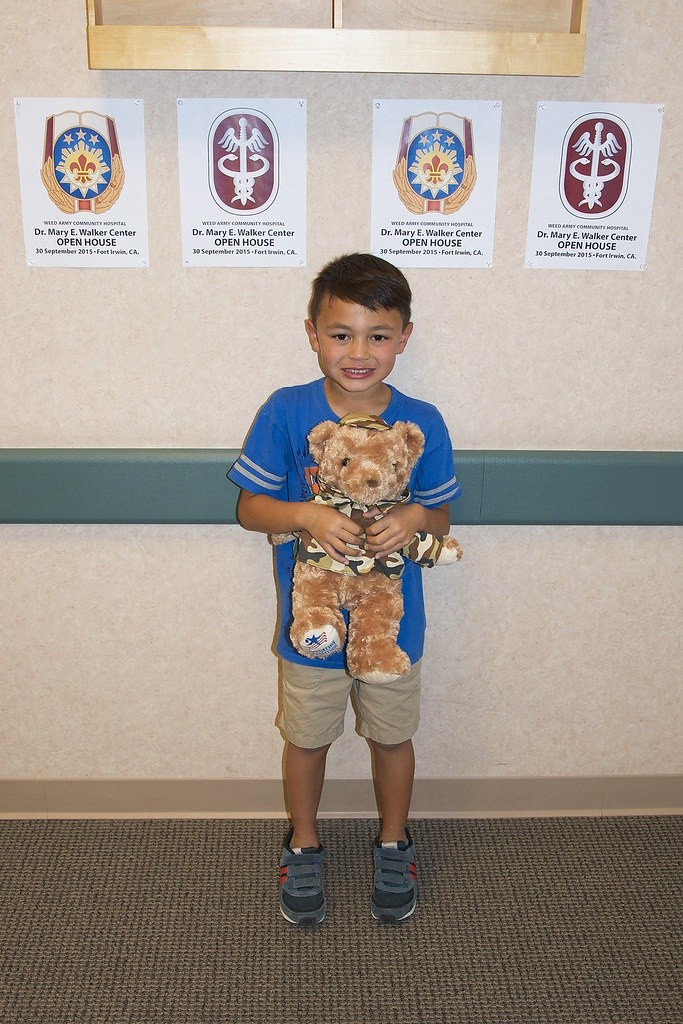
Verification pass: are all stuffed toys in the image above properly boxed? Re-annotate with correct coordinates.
[271,412,462,683]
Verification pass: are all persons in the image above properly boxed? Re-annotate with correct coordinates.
[227,253,461,924]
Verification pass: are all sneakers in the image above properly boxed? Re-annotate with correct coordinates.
[368,827,418,922]
[279,829,328,926]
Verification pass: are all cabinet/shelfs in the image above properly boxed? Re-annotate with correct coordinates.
[87,0,590,77]
